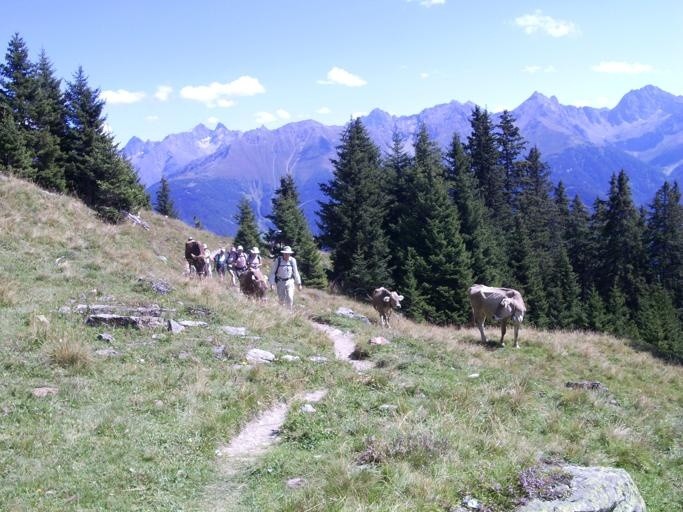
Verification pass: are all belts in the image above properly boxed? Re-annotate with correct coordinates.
[279,278,292,281]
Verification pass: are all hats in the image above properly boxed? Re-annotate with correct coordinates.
[280,246,295,253]
[203,244,260,254]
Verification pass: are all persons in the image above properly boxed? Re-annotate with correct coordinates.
[182,235,213,280]
[268,246,302,311]
[246,246,268,289]
[226,246,237,287]
[233,245,247,278]
[212,248,226,281]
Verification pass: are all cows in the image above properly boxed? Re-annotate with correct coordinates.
[372,287,403,327]
[183,241,207,279]
[239,268,268,301]
[466,283,526,349]
